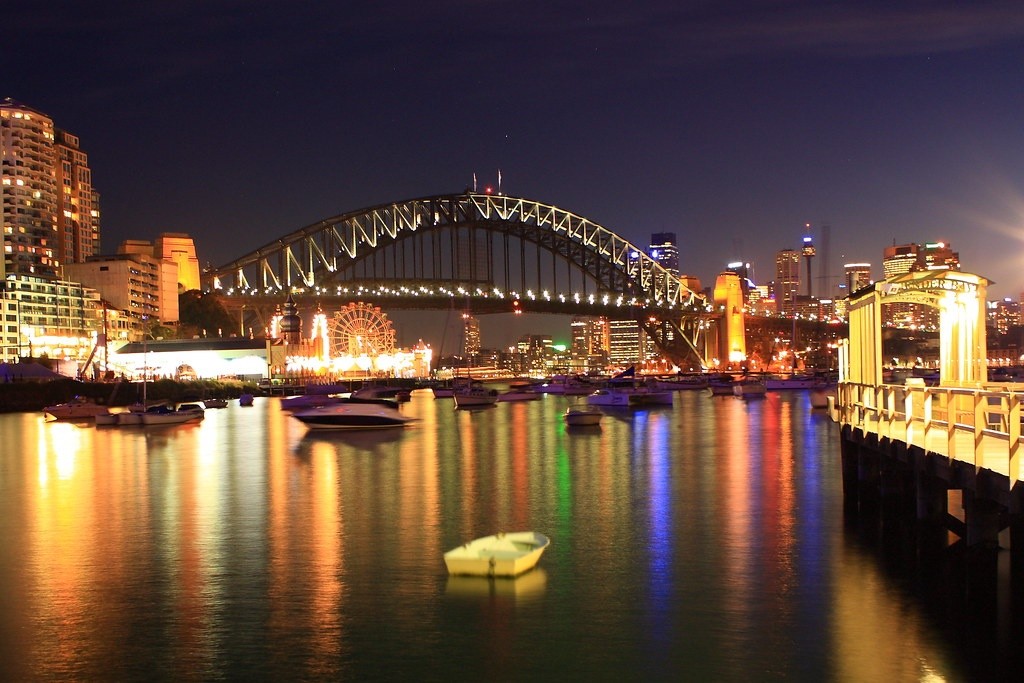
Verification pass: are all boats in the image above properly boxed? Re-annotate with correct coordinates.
[281,366,828,431]
[444,532,550,577]
[42,388,253,425]
